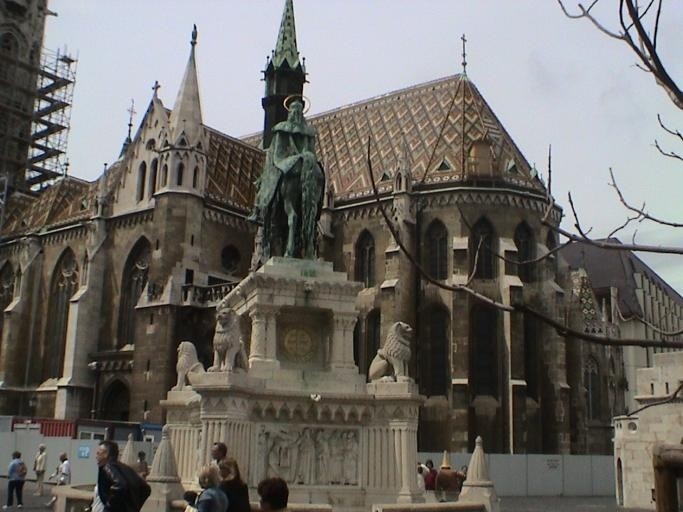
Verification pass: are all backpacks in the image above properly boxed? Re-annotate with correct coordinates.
[17,463,28,477]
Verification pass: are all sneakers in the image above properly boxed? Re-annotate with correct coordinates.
[2,504,13,510]
[16,503,23,509]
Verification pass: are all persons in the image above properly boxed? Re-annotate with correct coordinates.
[216,458,250,512]
[182,488,199,511]
[417,459,467,491]
[1,451,26,509]
[32,443,46,497]
[255,475,290,511]
[212,441,251,510]
[136,450,149,480]
[84,440,151,512]
[196,461,229,511]
[42,451,70,506]
